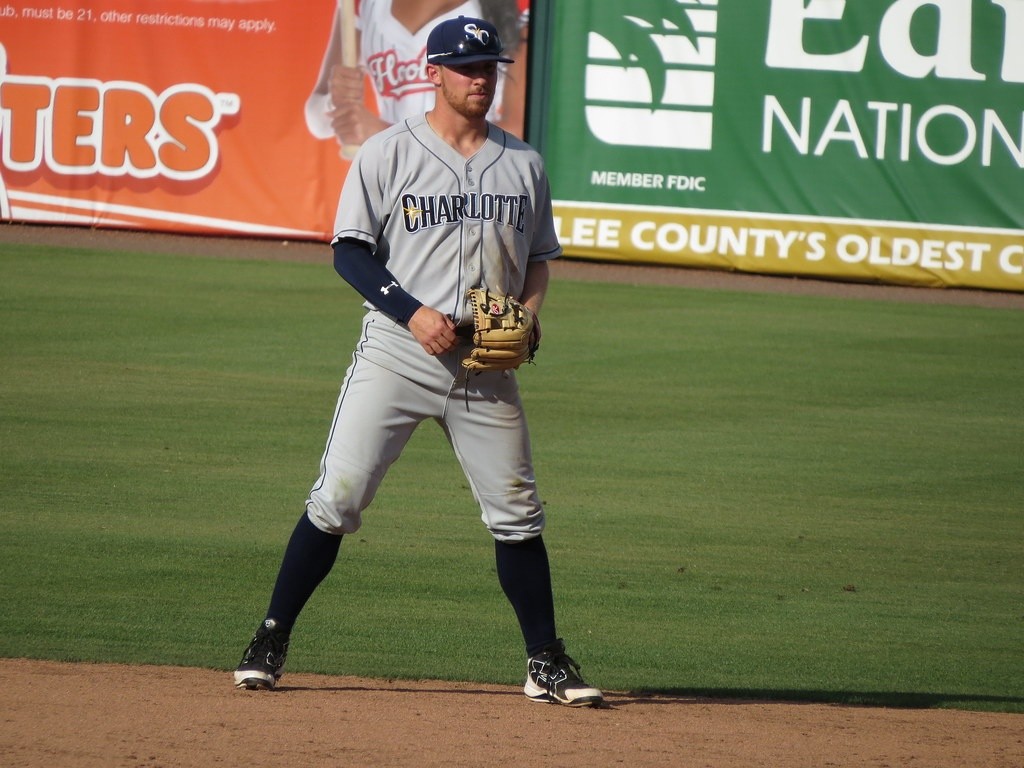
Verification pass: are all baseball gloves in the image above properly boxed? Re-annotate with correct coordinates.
[448,291,541,372]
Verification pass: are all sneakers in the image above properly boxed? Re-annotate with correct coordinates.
[233,619,290,691]
[523,637,603,707]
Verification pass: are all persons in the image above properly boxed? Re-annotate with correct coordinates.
[306,0,520,159]
[233,13,606,709]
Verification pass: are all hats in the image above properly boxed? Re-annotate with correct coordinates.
[426,16,515,66]
